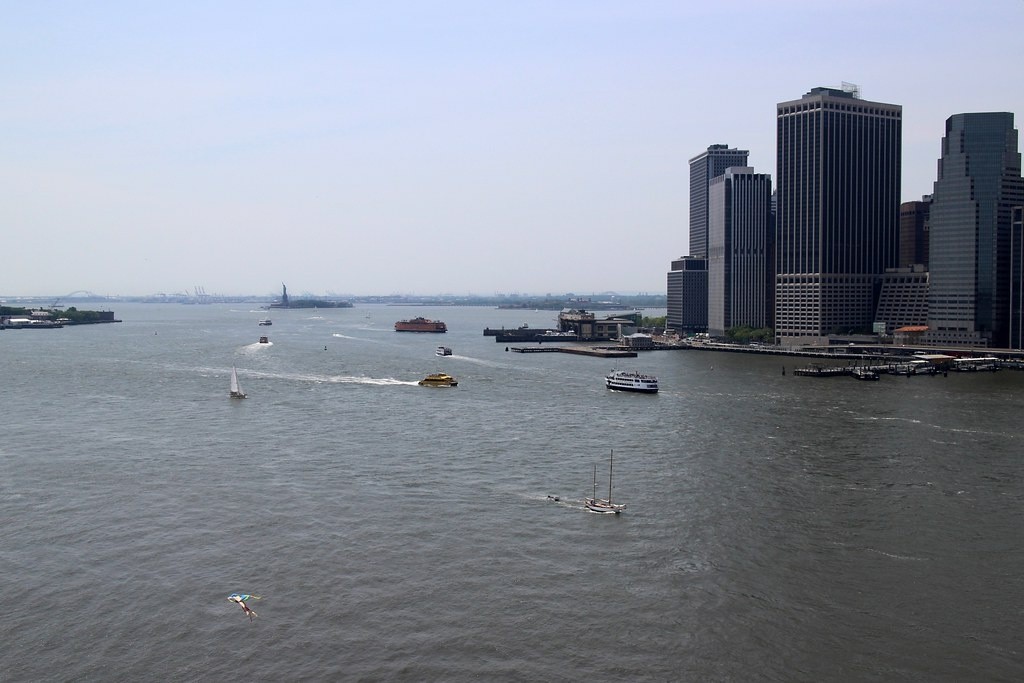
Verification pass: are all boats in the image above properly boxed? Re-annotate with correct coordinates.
[604,360,659,394]
[260,335,268,343]
[394,316,447,333]
[436,346,453,356]
[418,369,459,388]
[258,317,272,327]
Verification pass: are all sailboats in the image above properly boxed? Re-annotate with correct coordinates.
[229,364,248,400]
[587,448,627,516]
